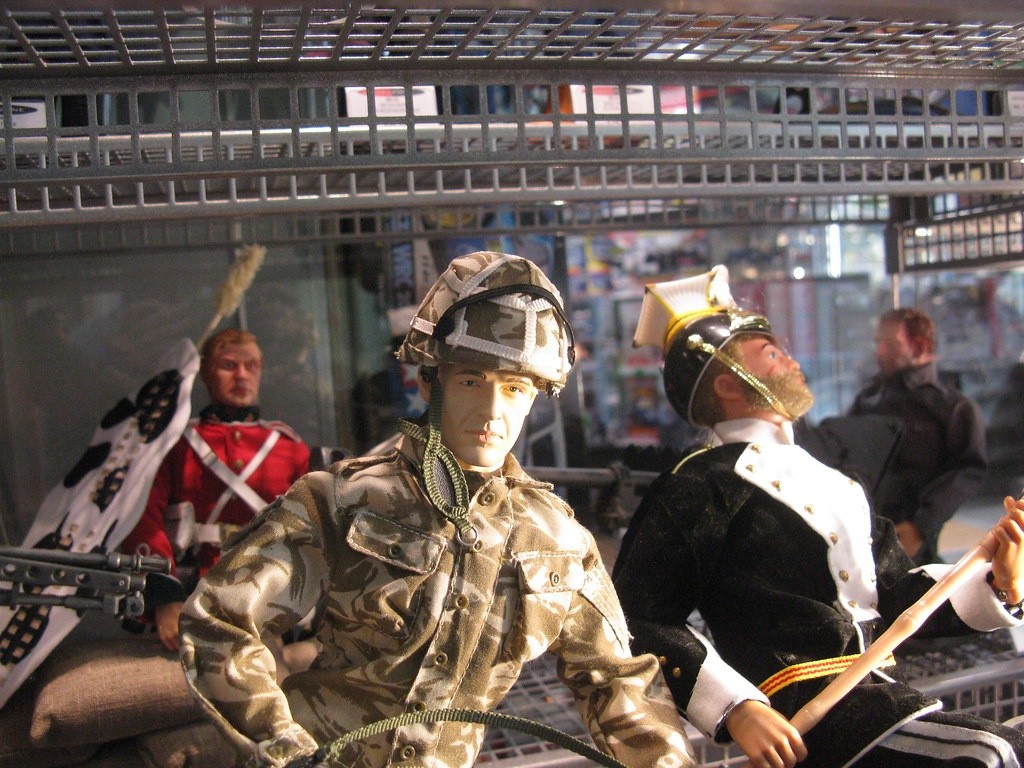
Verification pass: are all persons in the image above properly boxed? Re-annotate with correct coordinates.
[178,252,697,768]
[124,329,312,659]
[845,307,986,602]
[611,263,1024,768]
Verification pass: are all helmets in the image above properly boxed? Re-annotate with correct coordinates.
[633,264,777,429]
[395,251,575,398]
